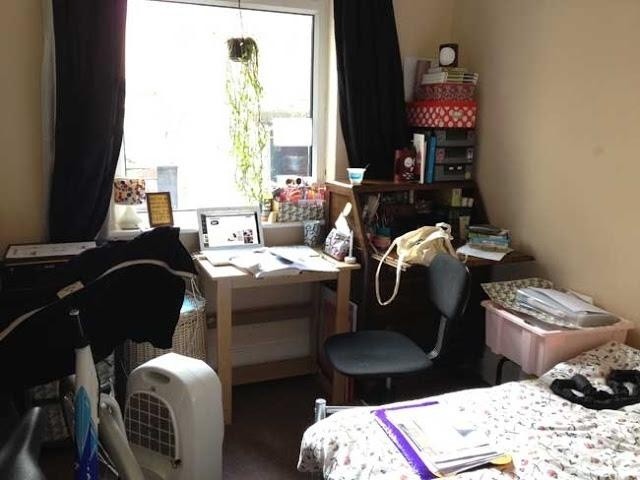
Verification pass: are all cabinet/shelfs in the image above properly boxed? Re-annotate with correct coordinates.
[425,128,478,182]
[1,237,118,453]
[324,171,537,286]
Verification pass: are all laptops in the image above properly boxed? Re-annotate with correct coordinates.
[197,206,265,266]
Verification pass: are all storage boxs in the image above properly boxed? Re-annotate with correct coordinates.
[479,298,636,379]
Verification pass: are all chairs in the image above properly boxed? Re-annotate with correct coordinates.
[322,251,473,401]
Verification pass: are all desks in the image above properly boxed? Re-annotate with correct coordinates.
[189,243,362,426]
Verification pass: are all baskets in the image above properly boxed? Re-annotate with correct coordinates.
[119,290,207,378]
[10,350,116,442]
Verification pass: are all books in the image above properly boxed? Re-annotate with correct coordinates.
[446,186,515,264]
[515,283,620,327]
[229,243,341,282]
[370,398,511,480]
[391,131,436,187]
[3,239,98,266]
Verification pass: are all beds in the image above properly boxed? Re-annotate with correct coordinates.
[296,338,640,479]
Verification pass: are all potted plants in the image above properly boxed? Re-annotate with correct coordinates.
[225,35,270,209]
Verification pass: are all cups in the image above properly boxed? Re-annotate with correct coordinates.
[303,220,322,248]
[346,167,366,186]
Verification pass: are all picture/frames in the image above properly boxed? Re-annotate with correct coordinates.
[143,190,174,227]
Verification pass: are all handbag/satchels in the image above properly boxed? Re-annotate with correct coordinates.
[396,226,448,266]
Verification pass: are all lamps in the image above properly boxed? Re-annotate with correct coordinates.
[114,176,146,229]
